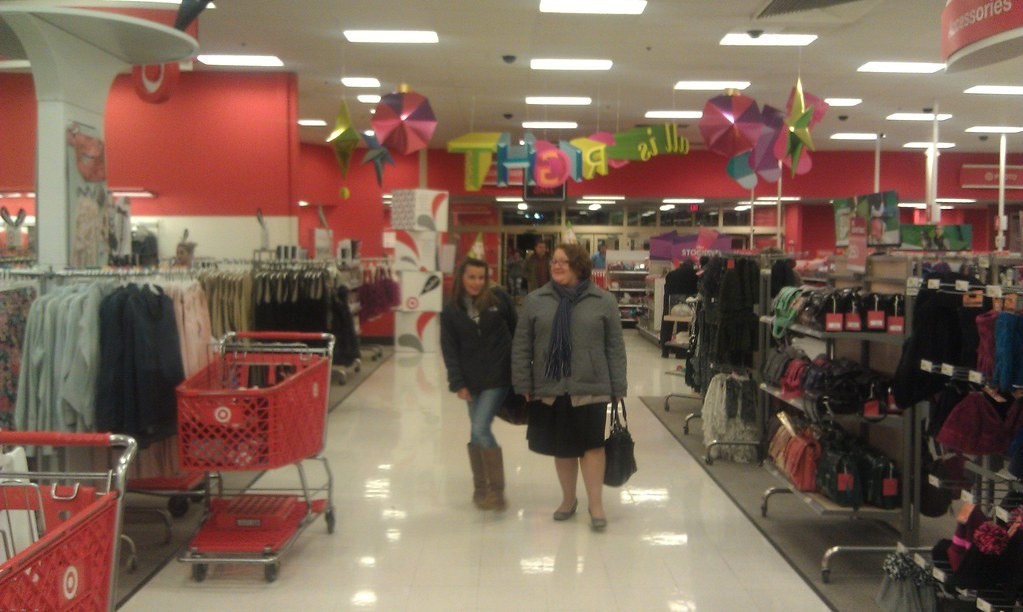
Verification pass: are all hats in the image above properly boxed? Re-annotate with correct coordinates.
[875,381,1023,612]
[682,259,697,266]
[892,330,948,410]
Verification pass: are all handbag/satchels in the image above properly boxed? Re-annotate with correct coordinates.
[762,284,905,511]
[603,396,637,487]
[495,388,531,425]
[361,265,401,320]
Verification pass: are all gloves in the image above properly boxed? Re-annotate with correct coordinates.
[992,311,1023,395]
[974,310,998,383]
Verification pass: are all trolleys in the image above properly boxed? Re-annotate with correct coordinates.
[0,432,142,612]
[175,331,337,582]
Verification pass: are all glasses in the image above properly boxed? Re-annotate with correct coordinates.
[549,258,569,267]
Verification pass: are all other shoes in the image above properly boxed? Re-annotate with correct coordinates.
[553,497,578,521]
[661,350,669,358]
[588,504,607,528]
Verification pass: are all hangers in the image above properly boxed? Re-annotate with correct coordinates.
[172,257,330,280]
[1,267,194,289]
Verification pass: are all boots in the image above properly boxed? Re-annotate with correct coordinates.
[511,296,515,305]
[515,295,523,306]
[480,447,505,511]
[466,443,488,509]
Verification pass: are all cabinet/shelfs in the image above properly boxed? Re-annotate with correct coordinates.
[605,250,704,356]
[759,255,934,580]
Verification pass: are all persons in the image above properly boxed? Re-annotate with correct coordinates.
[176,242,197,265]
[700,255,710,266]
[660,256,698,358]
[591,244,607,269]
[510,249,524,294]
[439,256,519,512]
[511,243,627,528]
[787,260,805,285]
[521,240,552,294]
